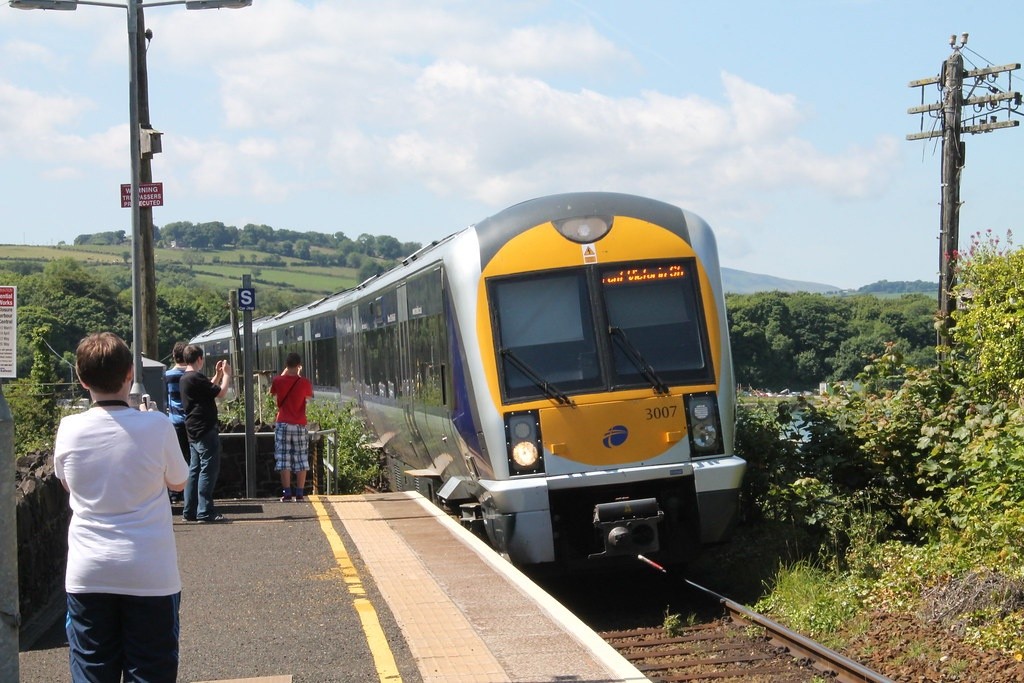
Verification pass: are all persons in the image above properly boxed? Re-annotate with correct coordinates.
[165,342,231,523]
[53,332,191,683]
[270,352,314,502]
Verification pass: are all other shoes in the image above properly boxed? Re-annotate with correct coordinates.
[281,494,292,502]
[296,494,304,501]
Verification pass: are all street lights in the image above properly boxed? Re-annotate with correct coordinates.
[7,1,256,408]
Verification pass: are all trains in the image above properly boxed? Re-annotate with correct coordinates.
[186,190,748,586]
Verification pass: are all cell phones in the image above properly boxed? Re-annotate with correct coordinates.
[142,394,150,410]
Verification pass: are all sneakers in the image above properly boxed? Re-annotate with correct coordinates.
[182,513,197,521]
[198,512,233,524]
